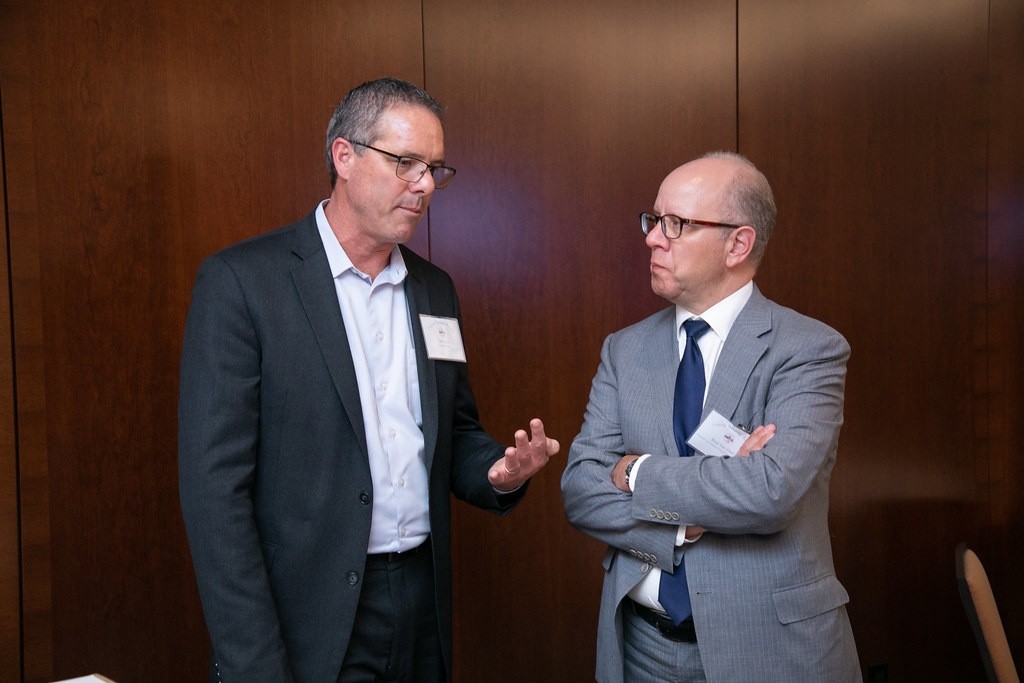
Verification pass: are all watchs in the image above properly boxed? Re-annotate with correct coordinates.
[625,458,638,485]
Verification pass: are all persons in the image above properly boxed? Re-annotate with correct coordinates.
[177,77,560,683]
[560,149,863,683]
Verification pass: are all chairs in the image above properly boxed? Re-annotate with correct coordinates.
[949,543,1024,683]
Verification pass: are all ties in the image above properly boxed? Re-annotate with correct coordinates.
[657,320,710,625]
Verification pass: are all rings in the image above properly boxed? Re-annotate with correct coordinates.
[504,468,521,475]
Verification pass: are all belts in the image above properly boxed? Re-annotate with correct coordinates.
[366,536,431,563]
[624,596,697,643]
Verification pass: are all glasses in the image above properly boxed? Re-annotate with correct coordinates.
[345,140,457,189]
[639,211,742,239]
